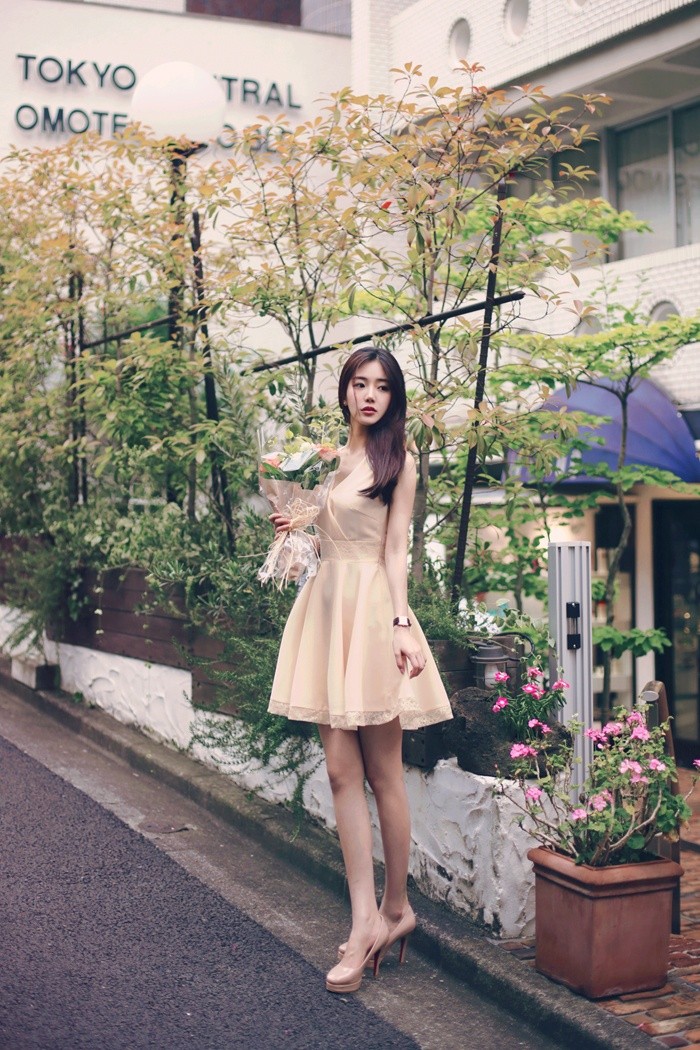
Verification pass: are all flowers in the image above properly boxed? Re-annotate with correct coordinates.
[485,668,700,867]
[0,489,565,845]
[259,412,343,490]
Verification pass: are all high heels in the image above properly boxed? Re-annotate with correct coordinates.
[339,905,416,964]
[325,914,390,992]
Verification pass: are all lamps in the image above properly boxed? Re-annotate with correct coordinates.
[469,640,509,692]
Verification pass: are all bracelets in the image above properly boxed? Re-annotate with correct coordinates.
[393,616,411,628]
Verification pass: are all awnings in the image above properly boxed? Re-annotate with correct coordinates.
[500,371,700,494]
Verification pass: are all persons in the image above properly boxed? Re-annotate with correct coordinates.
[268,346,454,993]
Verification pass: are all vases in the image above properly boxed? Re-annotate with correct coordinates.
[45,568,214,671]
[189,606,528,770]
[0,530,72,608]
[526,842,684,1000]
